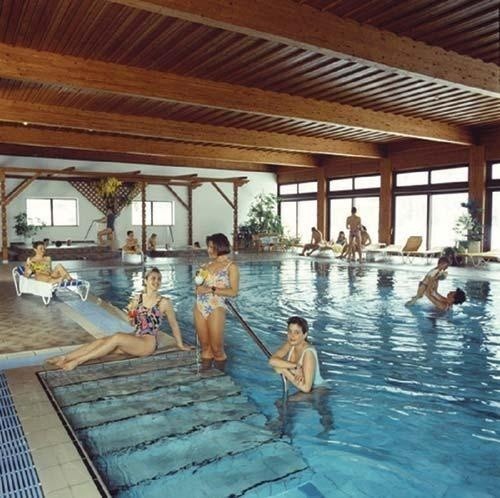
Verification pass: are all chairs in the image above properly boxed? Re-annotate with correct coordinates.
[250,229,499,270]
[9,262,94,306]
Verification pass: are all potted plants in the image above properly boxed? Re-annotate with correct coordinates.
[451,198,489,267]
[11,208,49,247]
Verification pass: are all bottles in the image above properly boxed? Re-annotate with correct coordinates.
[195,275,206,286]
[131,293,140,312]
[35,267,41,279]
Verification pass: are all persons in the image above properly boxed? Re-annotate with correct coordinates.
[297,206,374,259]
[42,267,198,373]
[43,238,49,247]
[93,206,115,250]
[148,233,158,252]
[191,233,240,373]
[267,315,340,441]
[123,230,145,261]
[424,275,468,327]
[403,256,450,309]
[23,240,76,283]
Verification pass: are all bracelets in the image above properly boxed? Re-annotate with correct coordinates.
[293,363,299,370]
[212,285,216,295]
